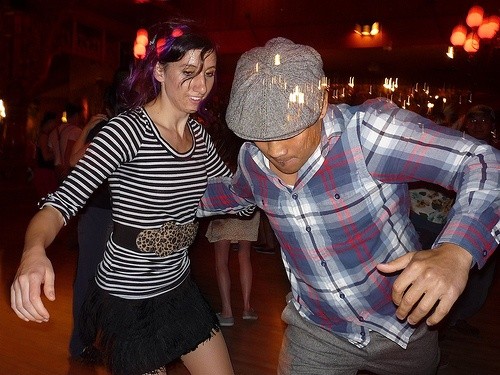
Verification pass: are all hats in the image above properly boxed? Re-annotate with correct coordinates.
[225,37,326,142]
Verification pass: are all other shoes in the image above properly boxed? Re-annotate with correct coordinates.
[82,345,104,361]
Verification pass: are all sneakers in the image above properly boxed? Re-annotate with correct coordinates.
[243,307,258,319]
[215,312,234,327]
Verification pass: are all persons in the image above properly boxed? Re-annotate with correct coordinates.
[0,87,277,364]
[10,17,234,375]
[404,101,500,338]
[195,36,500,375]
